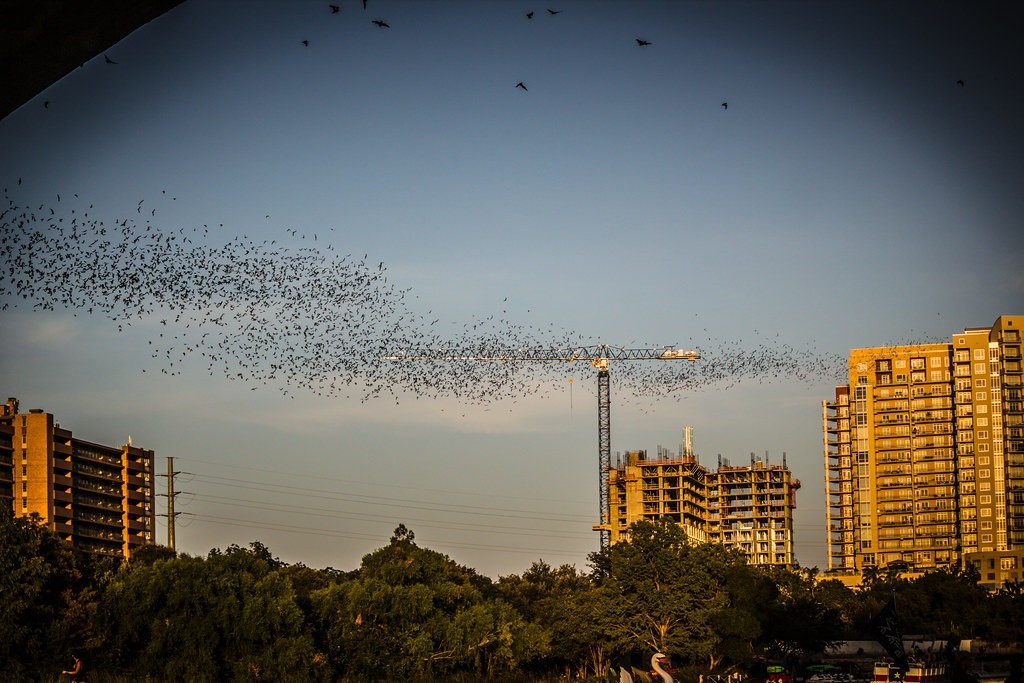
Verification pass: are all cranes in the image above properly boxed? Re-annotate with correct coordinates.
[381,341,703,562]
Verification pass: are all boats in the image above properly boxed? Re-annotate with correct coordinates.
[870,659,947,683]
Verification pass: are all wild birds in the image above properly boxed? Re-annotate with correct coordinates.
[41,0,730,108]
[0,176,955,420]
